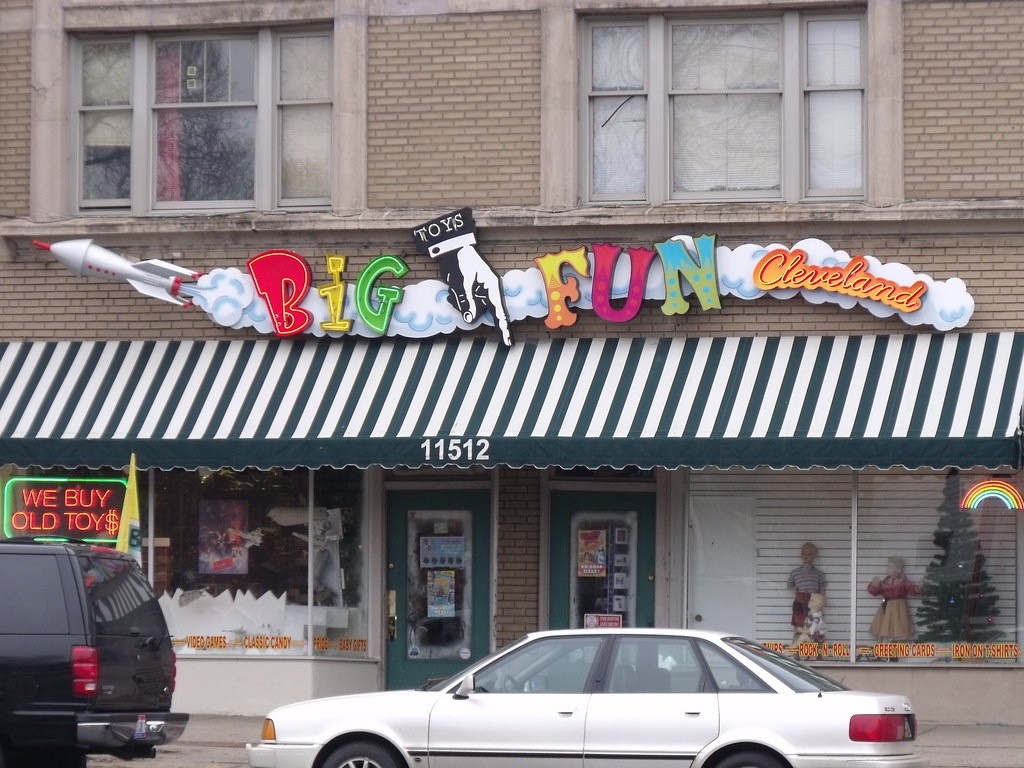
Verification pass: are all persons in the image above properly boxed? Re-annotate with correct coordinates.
[786,593,827,651]
[867,557,922,662]
[786,543,827,661]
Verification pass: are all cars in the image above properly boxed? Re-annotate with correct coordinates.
[246,628,926,768]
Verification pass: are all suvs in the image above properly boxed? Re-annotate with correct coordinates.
[0,536,192,768]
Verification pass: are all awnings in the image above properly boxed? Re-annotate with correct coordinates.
[0,332,1024,472]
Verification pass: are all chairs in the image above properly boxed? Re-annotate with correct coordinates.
[611,666,637,692]
[643,668,672,693]
